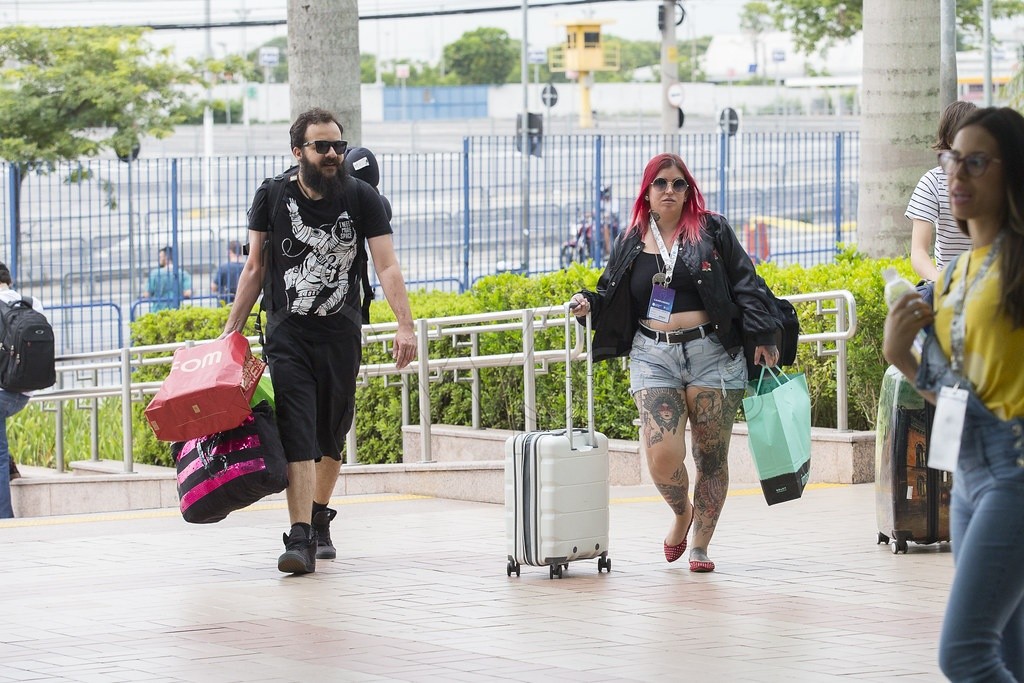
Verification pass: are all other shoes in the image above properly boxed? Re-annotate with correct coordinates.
[664,503,694,563]
[690,560,715,573]
[7,455,21,482]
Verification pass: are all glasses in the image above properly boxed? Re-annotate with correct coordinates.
[938,150,1002,179]
[302,141,348,154]
[650,178,689,193]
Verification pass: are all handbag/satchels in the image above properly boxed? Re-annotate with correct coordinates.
[144,333,289,524]
[744,277,799,381]
[742,364,811,506]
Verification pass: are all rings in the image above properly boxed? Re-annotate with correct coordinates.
[914,310,923,320]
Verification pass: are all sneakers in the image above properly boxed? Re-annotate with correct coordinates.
[311,509,337,560]
[278,525,317,573]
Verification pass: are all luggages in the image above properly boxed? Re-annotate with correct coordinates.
[505,301,611,580]
[875,364,954,553]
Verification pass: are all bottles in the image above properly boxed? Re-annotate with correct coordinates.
[882,266,928,354]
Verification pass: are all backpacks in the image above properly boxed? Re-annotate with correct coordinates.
[0,293,56,393]
[264,145,393,282]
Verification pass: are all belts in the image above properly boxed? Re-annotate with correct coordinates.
[636,321,715,343]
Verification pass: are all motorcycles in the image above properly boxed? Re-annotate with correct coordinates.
[559,186,624,272]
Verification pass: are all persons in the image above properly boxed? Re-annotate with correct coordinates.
[141,241,243,311]
[569,153,783,570]
[582,185,619,252]
[883,102,1024,683]
[0,262,45,518]
[220,111,419,572]
